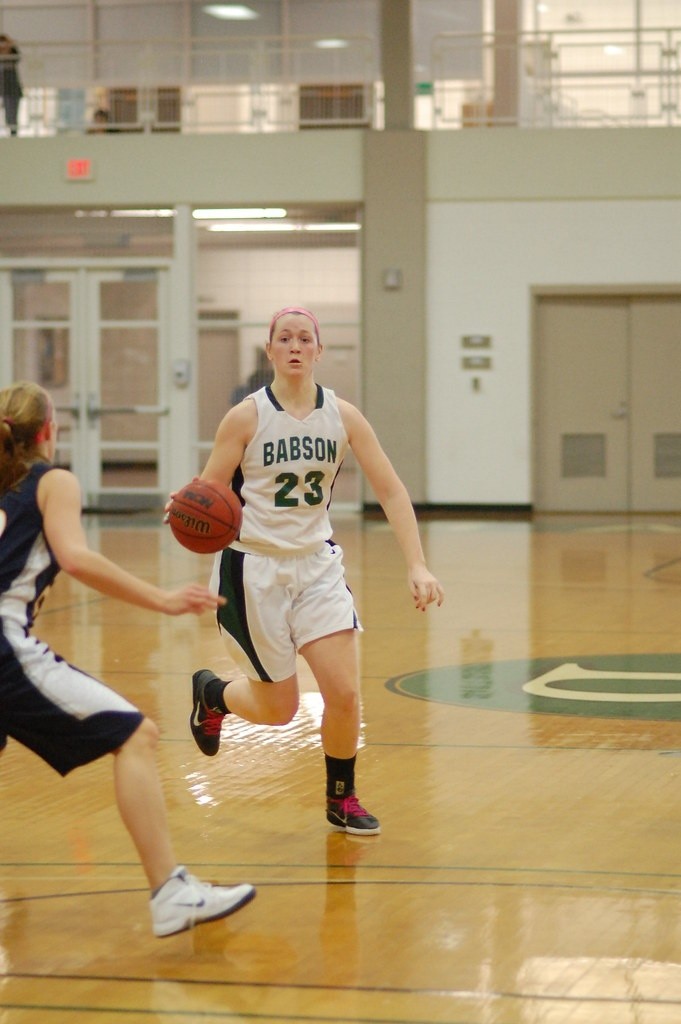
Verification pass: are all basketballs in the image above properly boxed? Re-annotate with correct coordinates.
[169,480,242,554]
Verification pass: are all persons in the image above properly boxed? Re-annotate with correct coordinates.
[0,35,23,135]
[0,383,258,936]
[163,308,442,837]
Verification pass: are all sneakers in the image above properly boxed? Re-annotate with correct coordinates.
[189,668,227,757]
[325,794,380,836]
[150,865,256,938]
